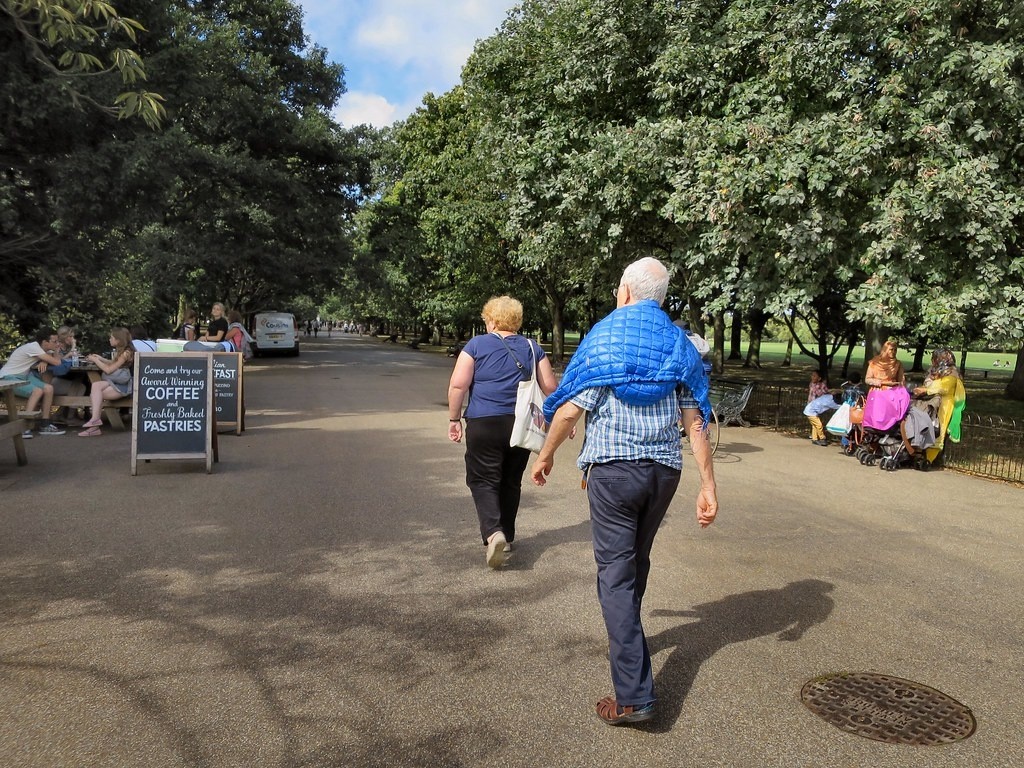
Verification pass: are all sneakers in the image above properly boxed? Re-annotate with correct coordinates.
[22,429,33,439]
[39,423,66,435]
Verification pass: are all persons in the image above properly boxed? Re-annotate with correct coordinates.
[860,341,905,456]
[808,370,828,438]
[447,296,578,568]
[992,359,1001,366]
[170,309,200,341]
[913,349,966,466]
[530,257,720,726]
[303,318,364,339]
[197,303,228,342]
[225,310,245,431]
[0,326,93,438]
[804,392,842,446]
[811,348,815,354]
[1004,361,1011,367]
[78,327,137,437]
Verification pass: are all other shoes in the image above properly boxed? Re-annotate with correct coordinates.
[83,418,103,427]
[78,428,102,436]
[486,532,506,568]
[503,542,511,552]
[812,440,820,445]
[820,439,828,446]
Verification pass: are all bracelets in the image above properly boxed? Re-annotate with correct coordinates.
[206,337,207,340]
[52,353,54,358]
[449,419,460,422]
[95,355,99,357]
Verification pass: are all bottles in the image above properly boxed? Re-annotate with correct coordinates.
[111,349,117,360]
[72,349,79,367]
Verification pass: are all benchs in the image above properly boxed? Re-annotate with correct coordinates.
[407,337,420,349]
[705,376,753,430]
[0,408,42,424]
[446,344,466,356]
[9,395,133,407]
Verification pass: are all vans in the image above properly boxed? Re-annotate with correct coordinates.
[252,313,300,358]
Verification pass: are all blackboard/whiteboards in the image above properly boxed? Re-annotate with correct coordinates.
[213,352,244,425]
[131,352,218,459]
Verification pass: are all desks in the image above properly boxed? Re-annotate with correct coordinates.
[30,352,127,432]
[0,378,31,465]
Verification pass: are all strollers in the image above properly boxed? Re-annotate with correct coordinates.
[840,380,941,473]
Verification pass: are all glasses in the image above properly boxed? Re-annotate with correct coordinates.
[613,284,626,298]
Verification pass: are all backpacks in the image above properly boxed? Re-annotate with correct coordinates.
[172,323,191,339]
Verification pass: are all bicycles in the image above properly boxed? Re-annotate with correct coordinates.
[677,385,719,456]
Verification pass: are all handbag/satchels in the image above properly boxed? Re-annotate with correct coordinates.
[102,368,132,397]
[509,338,546,456]
[826,397,855,437]
[849,395,866,424]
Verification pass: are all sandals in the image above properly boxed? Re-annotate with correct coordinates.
[595,697,657,726]
